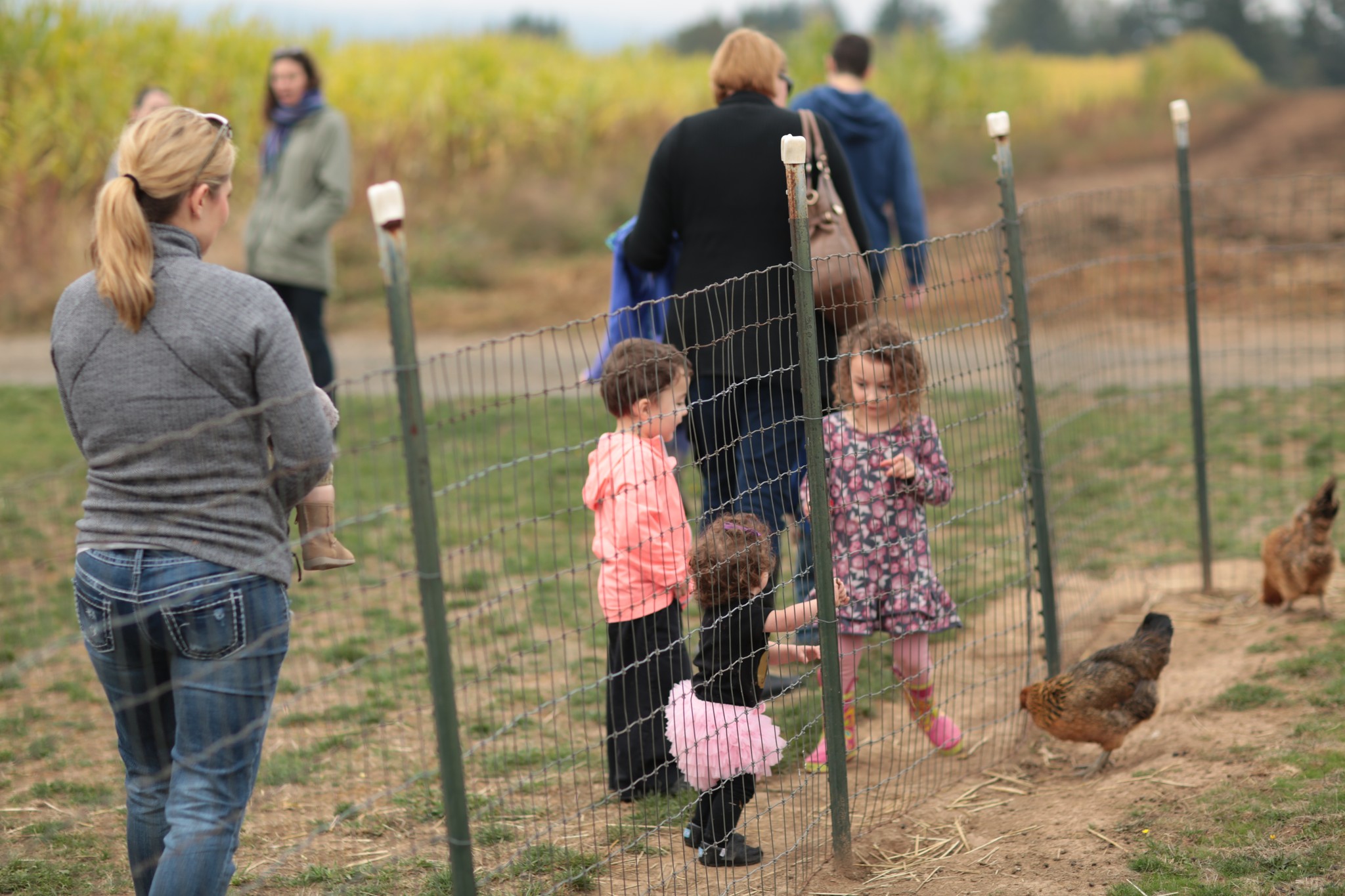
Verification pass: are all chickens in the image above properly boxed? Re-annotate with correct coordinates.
[1018,612,1174,773]
[1261,475,1341,618]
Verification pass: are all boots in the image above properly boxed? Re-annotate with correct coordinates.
[294,485,357,570]
[891,663,967,755]
[804,667,856,772]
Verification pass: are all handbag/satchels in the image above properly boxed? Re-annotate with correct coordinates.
[797,106,875,332]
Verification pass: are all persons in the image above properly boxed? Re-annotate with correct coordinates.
[664,510,851,871]
[581,335,698,803]
[783,29,931,318]
[104,85,176,186]
[620,20,880,703]
[242,49,343,456]
[799,318,964,775]
[48,105,340,894]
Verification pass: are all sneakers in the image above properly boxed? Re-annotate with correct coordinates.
[684,823,745,846]
[697,839,764,867]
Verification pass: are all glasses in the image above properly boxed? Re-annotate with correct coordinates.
[186,109,232,194]
[777,73,796,96]
